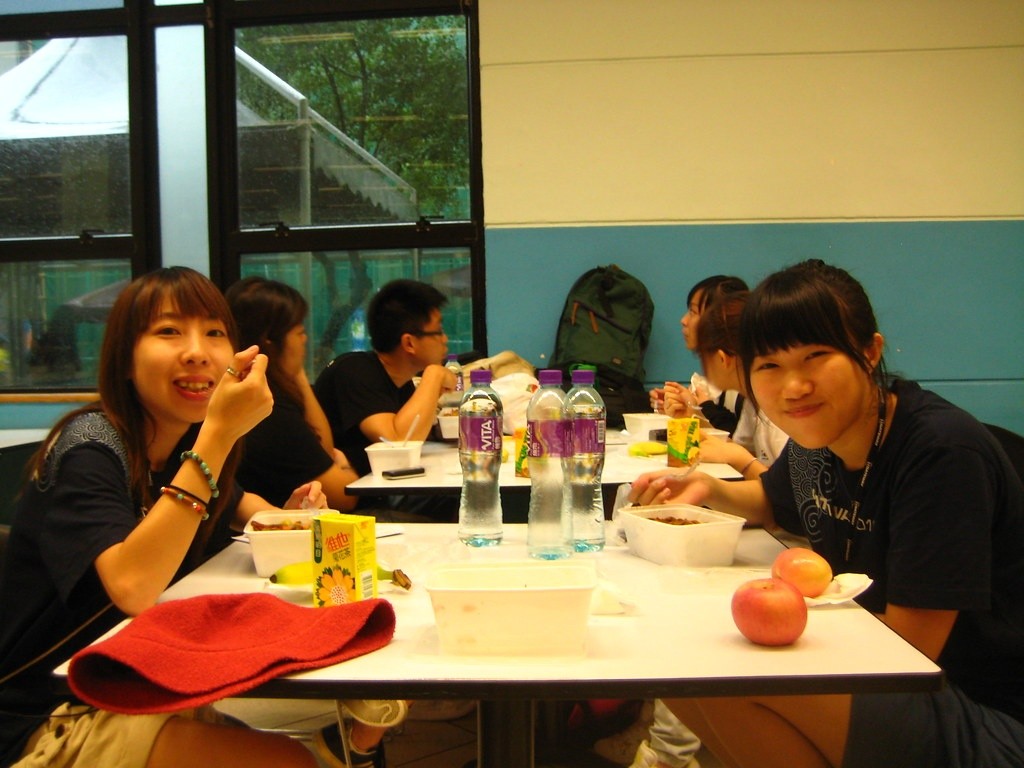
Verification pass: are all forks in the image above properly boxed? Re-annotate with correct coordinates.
[650,459,700,480]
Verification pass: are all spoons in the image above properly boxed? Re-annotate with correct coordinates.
[679,395,703,410]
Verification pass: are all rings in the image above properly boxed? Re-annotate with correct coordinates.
[226,366,240,378]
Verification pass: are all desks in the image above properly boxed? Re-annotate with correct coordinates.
[0,427,55,524]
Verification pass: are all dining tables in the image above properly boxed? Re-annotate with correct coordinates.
[51,521,943,768]
[344,428,746,768]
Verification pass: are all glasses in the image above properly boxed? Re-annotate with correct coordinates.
[421,328,445,343]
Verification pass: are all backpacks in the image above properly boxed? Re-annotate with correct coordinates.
[534,362,654,429]
[551,264,654,385]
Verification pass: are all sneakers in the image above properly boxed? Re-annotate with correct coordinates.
[312,718,386,768]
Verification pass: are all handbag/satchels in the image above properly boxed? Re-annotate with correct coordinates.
[412,350,535,397]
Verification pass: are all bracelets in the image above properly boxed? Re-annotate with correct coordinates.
[179,447,220,498]
[168,485,209,506]
[341,464,353,470]
[741,458,757,474]
[159,486,209,520]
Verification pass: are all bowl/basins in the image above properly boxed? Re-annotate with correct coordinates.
[243,510,340,578]
[364,441,424,474]
[617,504,747,568]
[622,413,670,441]
[424,563,595,657]
[436,407,459,439]
[700,427,730,443]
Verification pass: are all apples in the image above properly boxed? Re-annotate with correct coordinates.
[731,578,807,645]
[771,547,832,597]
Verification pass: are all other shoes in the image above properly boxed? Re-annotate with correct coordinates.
[628,740,700,768]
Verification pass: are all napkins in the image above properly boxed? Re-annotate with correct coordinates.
[803,573,874,607]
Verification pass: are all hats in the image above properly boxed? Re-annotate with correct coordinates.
[67,593,397,714]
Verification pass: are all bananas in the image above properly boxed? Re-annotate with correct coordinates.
[627,442,668,457]
[269,557,412,590]
[501,449,508,461]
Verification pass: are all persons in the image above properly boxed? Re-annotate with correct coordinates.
[310,279,462,522]
[0,265,412,768]
[598,260,1024,768]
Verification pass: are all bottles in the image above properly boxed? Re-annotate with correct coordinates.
[445,355,463,391]
[561,371,604,553]
[527,370,576,561]
[458,370,503,547]
